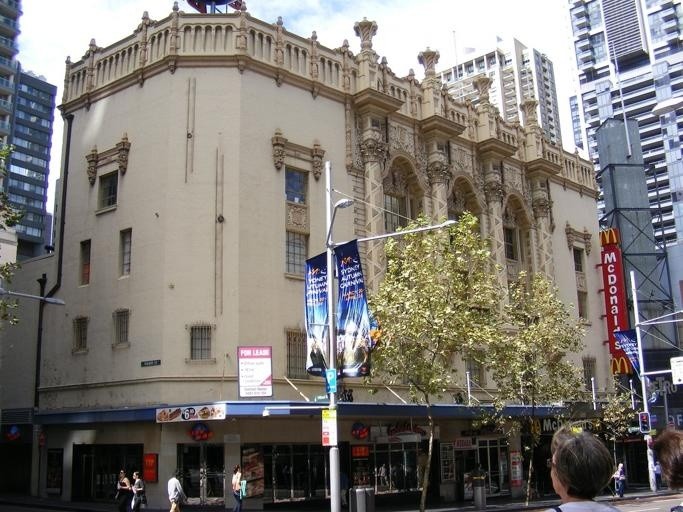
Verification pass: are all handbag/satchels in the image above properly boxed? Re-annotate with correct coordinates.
[140,494,147,504]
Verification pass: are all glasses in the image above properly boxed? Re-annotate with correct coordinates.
[120,471,126,473]
[546,458,557,469]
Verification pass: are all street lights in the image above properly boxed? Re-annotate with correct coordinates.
[321,161,458,512]
[0,287,66,307]
[651,96,682,116]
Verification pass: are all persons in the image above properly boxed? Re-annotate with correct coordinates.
[231,465,243,511]
[613,463,626,497]
[167,471,187,512]
[652,430,683,511]
[378,463,397,487]
[651,460,662,490]
[543,431,626,512]
[115,469,146,512]
[206,464,219,497]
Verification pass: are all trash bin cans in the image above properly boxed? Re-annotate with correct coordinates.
[349,485,375,512]
[473,477,486,510]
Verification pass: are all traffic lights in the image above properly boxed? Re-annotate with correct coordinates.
[638,411,651,434]
